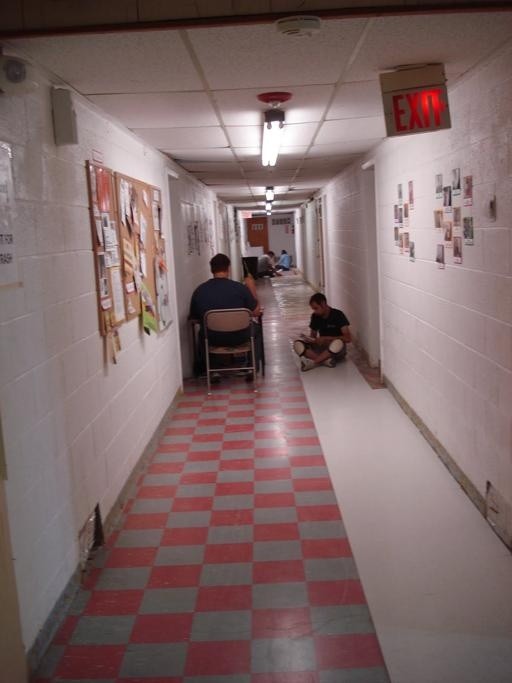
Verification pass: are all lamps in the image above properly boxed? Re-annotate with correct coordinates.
[256,92,293,167]
[265,186,274,216]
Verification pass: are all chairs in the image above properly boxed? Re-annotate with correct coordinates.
[204,308,257,395]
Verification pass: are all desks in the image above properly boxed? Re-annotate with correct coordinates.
[187,307,265,377]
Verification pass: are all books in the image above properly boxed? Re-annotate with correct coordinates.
[299,333,315,342]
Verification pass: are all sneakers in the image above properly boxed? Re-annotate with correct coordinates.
[301,359,316,371]
[323,356,336,367]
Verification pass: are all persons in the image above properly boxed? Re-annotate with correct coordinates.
[274,250,290,271]
[258,251,283,278]
[293,292,352,371]
[189,252,261,384]
